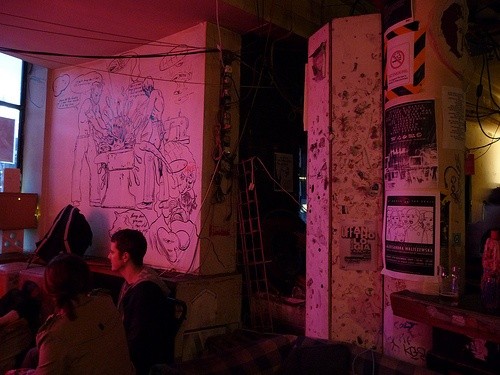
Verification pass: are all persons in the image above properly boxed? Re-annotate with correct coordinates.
[480,229,500,291]
[108,228,175,375]
[23,253,136,375]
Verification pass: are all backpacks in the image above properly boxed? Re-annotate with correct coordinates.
[25,203,93,270]
